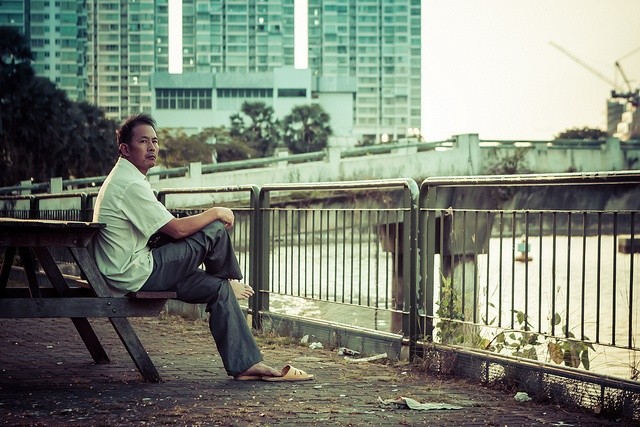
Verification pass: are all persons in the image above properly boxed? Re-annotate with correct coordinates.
[90,114,315,380]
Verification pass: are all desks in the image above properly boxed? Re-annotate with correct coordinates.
[1,219,166,384]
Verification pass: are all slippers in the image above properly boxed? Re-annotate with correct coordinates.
[262,365,315,381]
[235,367,283,382]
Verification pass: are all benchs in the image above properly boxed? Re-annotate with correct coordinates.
[78,278,177,383]
